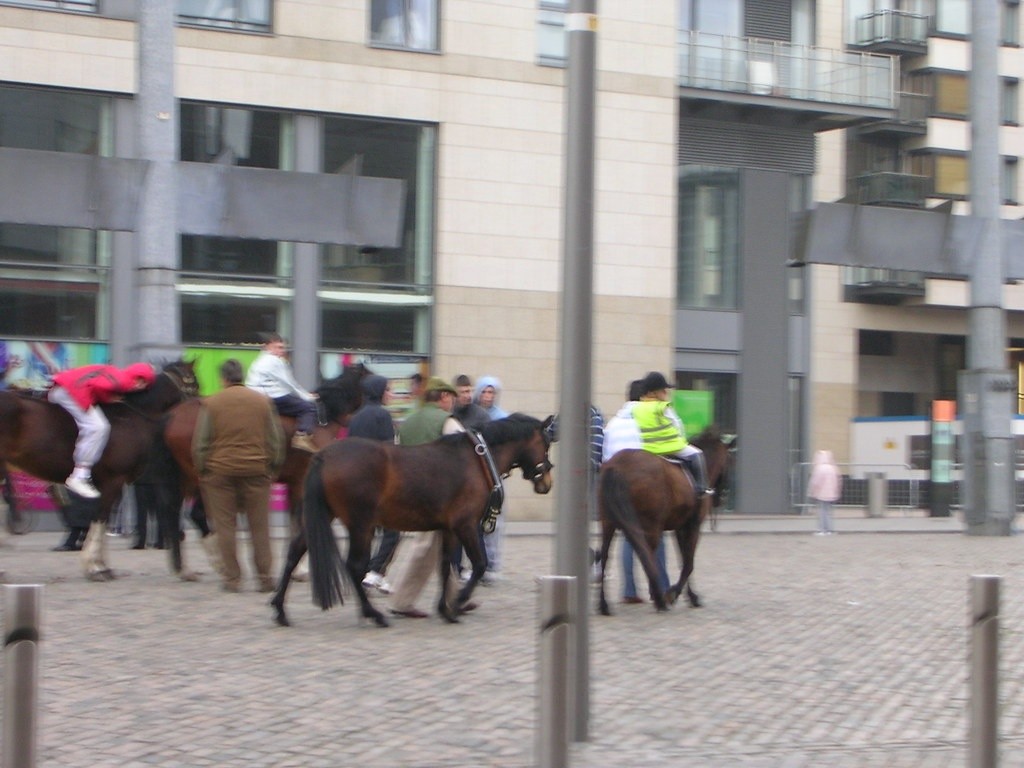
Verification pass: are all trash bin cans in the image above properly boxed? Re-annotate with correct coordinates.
[866,472,888,520]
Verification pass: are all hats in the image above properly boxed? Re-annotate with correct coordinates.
[642,373,673,392]
[425,377,458,397]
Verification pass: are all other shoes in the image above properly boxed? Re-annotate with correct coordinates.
[626,596,643,603]
[291,434,317,455]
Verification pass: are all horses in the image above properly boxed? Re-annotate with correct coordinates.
[152,360,393,581]
[596,426,738,615]
[0,359,200,581]
[270,411,553,628]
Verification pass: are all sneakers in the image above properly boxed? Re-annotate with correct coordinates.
[362,571,393,594]
[66,475,100,498]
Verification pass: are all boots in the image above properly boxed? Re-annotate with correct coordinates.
[692,453,714,498]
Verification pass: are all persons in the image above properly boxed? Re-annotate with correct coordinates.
[550,403,604,567]
[601,382,671,612]
[190,360,282,591]
[348,375,393,588]
[410,374,424,398]
[105,484,136,536]
[247,330,322,453]
[471,376,509,587]
[449,373,493,571]
[47,363,155,500]
[631,372,716,495]
[386,378,478,619]
[807,451,843,537]
